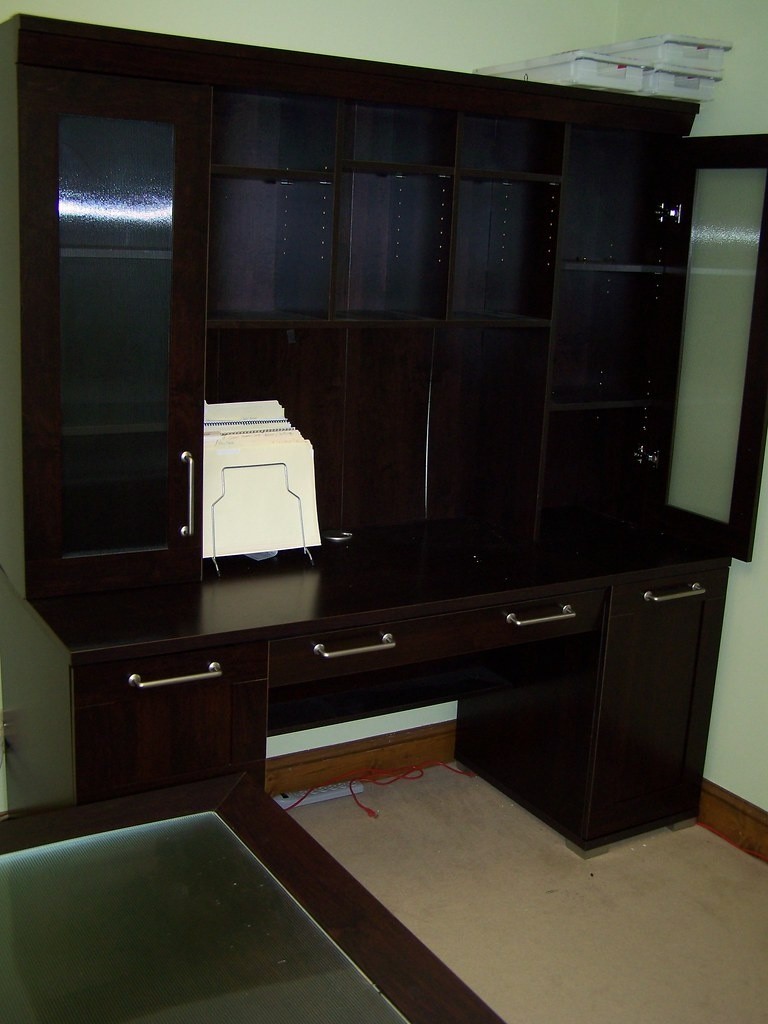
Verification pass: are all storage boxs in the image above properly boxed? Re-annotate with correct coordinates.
[473,33,732,101]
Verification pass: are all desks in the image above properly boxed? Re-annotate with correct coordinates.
[0,771,505,1024]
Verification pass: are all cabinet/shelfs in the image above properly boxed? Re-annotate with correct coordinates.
[1,14,768,602]
[0,509,730,852]
